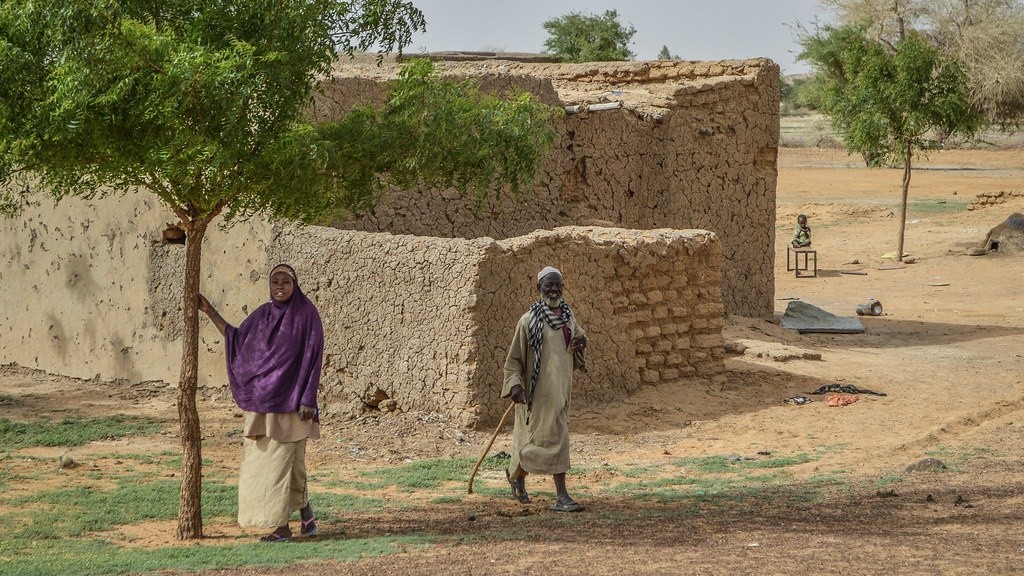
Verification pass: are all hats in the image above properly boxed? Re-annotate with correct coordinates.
[537,266,562,283]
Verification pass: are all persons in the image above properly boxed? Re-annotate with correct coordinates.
[791,214,812,249]
[196,263,325,542]
[500,265,584,514]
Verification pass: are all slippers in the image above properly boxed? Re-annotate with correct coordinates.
[506,469,530,504]
[550,495,586,513]
[300,513,316,537]
[265,534,292,543]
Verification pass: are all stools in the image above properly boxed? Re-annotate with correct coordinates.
[787,241,818,279]
